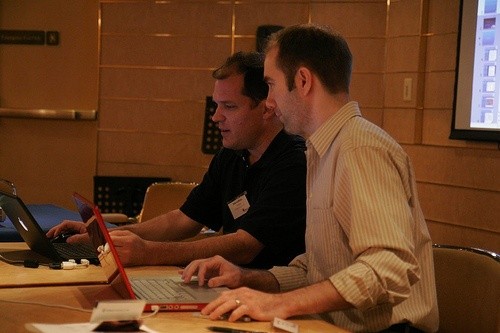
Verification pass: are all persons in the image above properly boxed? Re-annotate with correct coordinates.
[46,51,307,270]
[182,25,439,333]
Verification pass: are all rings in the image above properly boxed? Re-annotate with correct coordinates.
[234,299,240,307]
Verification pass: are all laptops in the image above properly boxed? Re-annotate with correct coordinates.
[0,191,100,264]
[72,192,231,313]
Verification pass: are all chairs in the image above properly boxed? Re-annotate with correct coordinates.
[432,244,500,333]
[102,182,199,241]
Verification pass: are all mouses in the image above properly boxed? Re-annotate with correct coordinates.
[51,232,75,244]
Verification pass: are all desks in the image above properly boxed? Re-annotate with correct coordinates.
[0,203,120,242]
[0,242,352,333]
